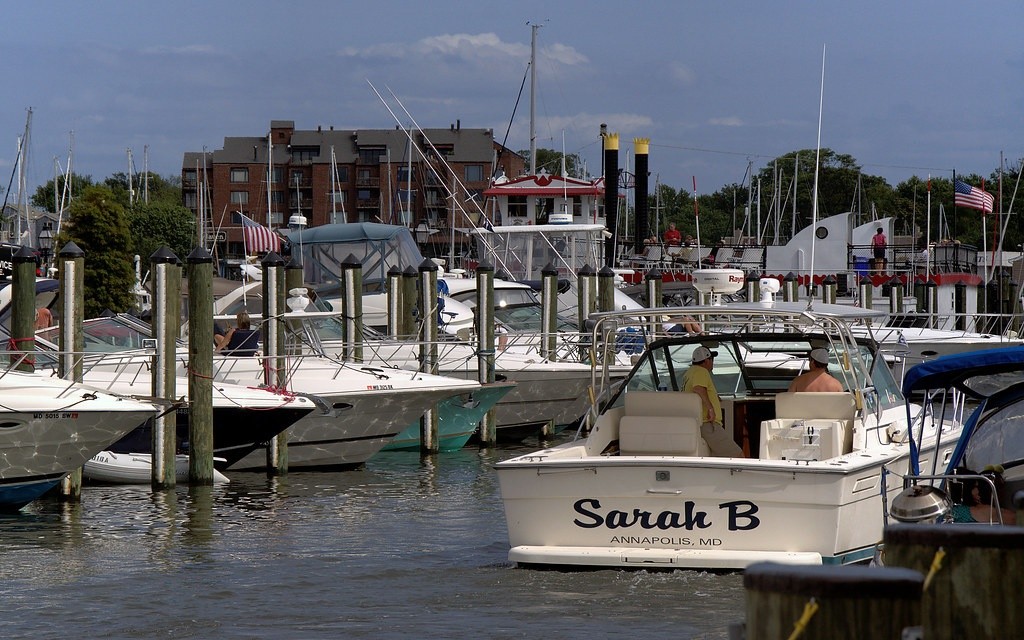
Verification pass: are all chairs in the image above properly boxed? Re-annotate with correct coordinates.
[222,330,260,355]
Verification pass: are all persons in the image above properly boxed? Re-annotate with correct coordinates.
[662,315,705,337]
[35,300,53,351]
[914,244,928,262]
[937,469,1017,524]
[681,346,747,458]
[214,312,250,351]
[787,349,844,393]
[871,228,887,276]
[643,222,694,272]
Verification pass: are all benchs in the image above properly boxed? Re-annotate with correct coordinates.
[776,393,857,452]
[619,391,713,457]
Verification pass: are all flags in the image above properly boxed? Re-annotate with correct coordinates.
[955,177,994,213]
[242,217,281,252]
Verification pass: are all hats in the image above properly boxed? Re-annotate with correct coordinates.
[692,346,719,362]
[810,348,831,365]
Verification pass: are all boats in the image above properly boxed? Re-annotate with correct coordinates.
[495,41,967,569]
[0,18,1024,485]
[747,346,1024,640]
